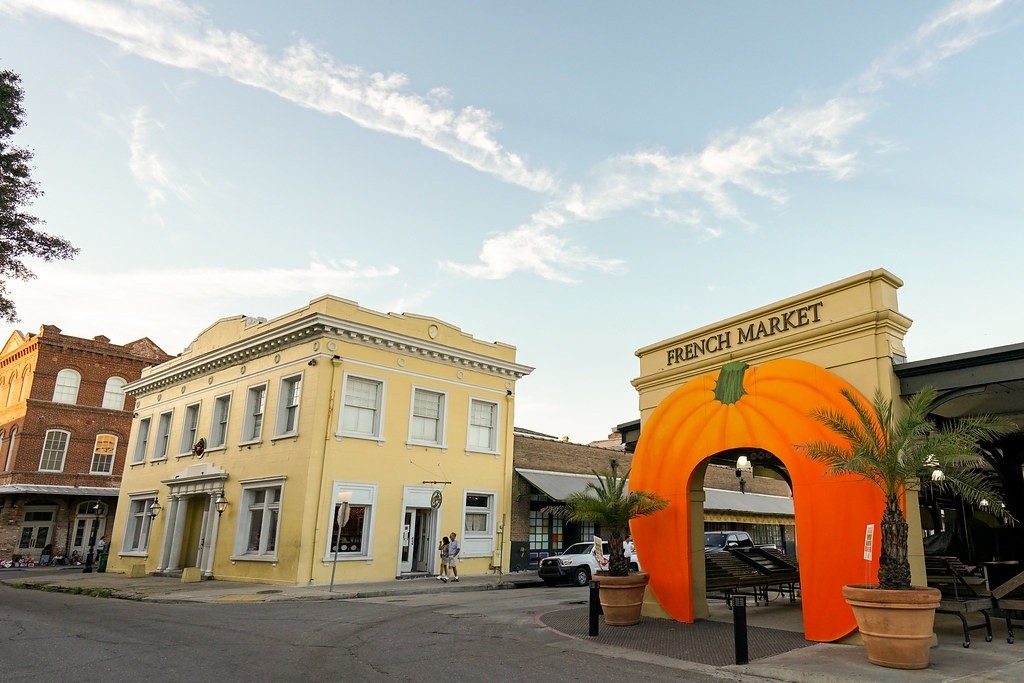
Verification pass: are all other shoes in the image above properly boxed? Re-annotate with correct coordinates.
[436,576,442,580]
[451,577,459,582]
[440,577,447,583]
[442,576,449,579]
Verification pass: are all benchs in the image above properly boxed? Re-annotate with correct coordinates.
[924,553,996,649]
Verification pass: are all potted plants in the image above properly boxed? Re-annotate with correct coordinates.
[800,385,1016,672]
[543,458,670,626]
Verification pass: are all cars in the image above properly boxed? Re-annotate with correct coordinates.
[704,530,755,552]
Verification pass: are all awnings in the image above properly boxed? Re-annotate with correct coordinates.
[515,467,794,516]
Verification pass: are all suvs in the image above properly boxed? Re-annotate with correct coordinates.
[538,541,642,588]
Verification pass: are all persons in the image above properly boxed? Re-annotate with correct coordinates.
[436,537,450,580]
[71,550,81,566]
[622,536,635,570]
[440,532,460,581]
[95,536,106,564]
[51,551,64,565]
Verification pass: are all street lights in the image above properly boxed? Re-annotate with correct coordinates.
[82,500,106,573]
